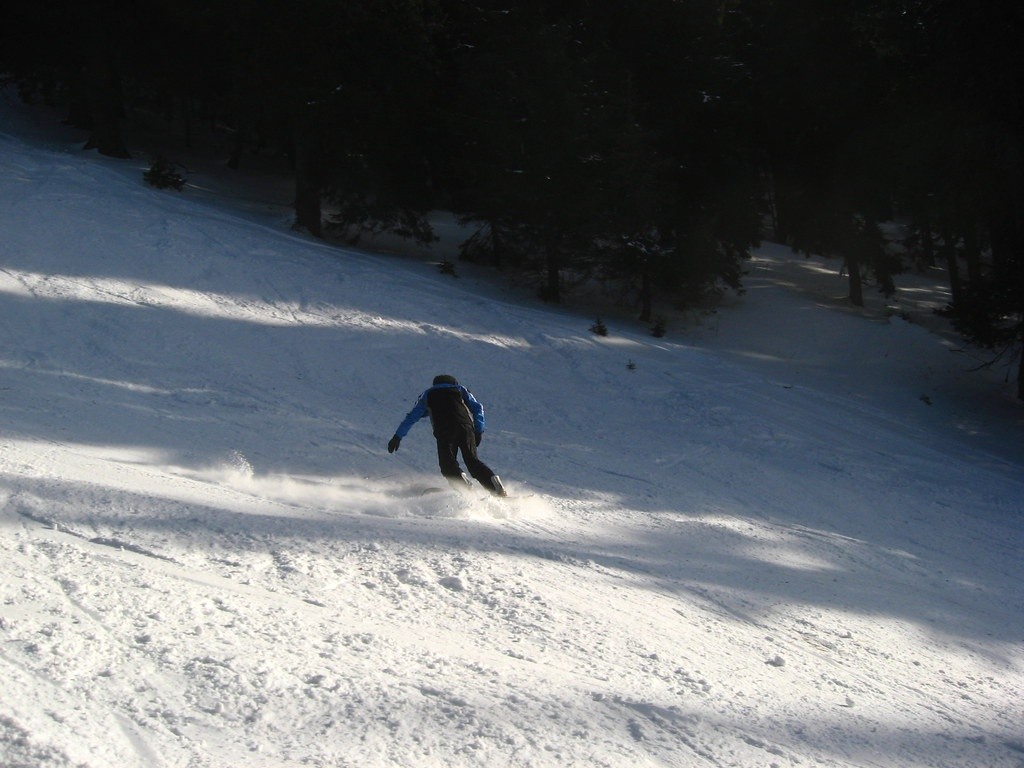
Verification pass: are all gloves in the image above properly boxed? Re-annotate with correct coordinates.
[388,434,400,453]
[475,432,482,447]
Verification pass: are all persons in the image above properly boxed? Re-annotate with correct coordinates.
[387,375,506,496]
